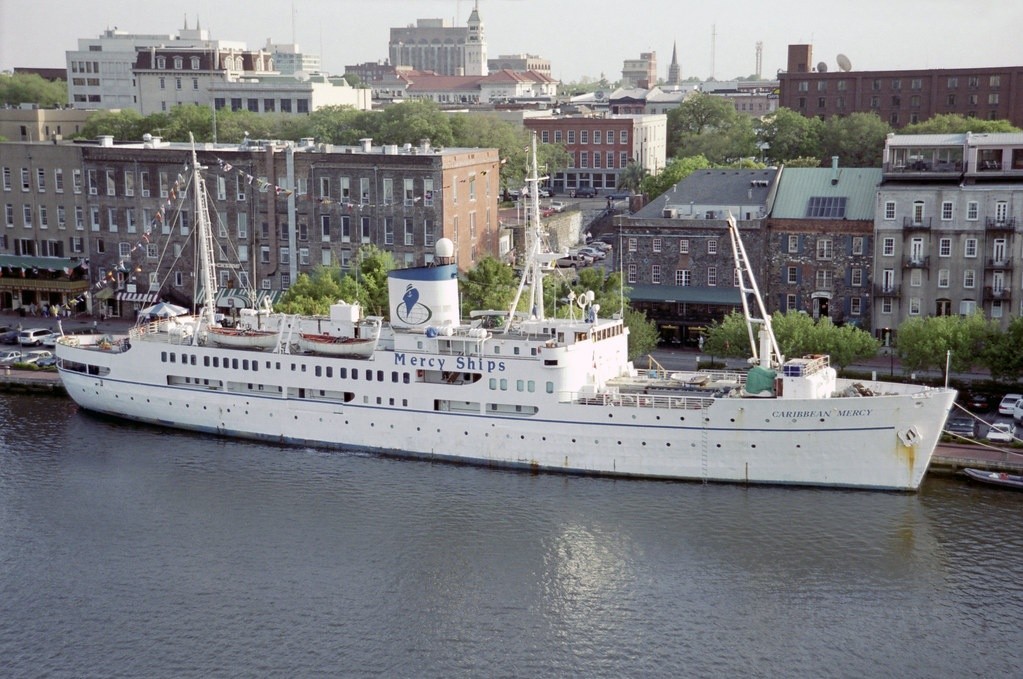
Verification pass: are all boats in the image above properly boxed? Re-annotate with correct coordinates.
[295,332,377,360]
[207,324,281,348]
[670,372,710,386]
[963,468,1023,490]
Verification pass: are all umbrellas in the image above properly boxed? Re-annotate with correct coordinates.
[143,302,189,318]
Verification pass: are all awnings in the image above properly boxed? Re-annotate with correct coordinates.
[194,285,292,311]
[117,292,159,303]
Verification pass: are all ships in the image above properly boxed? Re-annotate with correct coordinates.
[53,130,962,495]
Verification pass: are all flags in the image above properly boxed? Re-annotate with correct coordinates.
[0,265,74,278]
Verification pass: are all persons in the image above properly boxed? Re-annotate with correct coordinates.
[697,335,704,352]
[31,303,51,318]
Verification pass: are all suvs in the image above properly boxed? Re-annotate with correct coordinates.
[17,328,54,347]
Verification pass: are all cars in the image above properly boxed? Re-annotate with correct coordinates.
[35,353,56,367]
[998,394,1023,415]
[0,350,27,366]
[568,186,598,198]
[557,241,612,269]
[604,189,635,200]
[1013,399,1023,427]
[985,421,1017,443]
[967,390,993,413]
[499,186,566,217]
[949,416,980,440]
[22,349,52,366]
[0,326,20,344]
